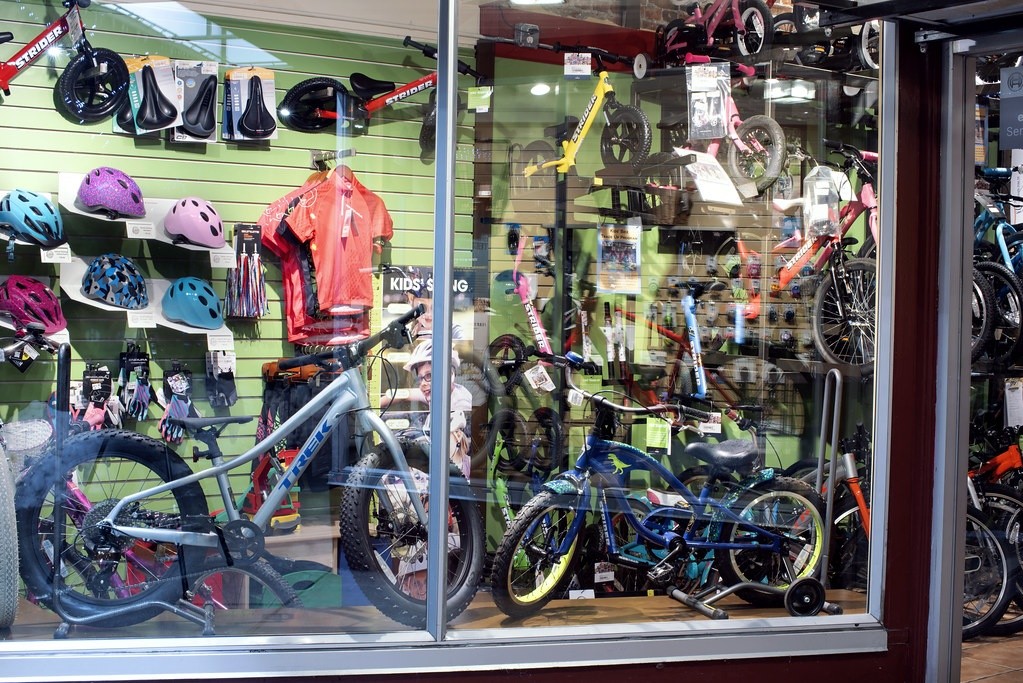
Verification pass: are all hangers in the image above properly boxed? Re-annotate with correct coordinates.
[307,150,353,183]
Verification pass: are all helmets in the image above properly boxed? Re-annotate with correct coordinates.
[404,265,433,298]
[422,411,467,437]
[398,539,428,577]
[80,252,149,310]
[0,274,67,338]
[0,188,68,263]
[403,339,460,372]
[161,276,224,329]
[77,166,146,220]
[164,196,225,248]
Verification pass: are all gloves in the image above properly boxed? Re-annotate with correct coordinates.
[206,350,238,408]
[69,403,87,422]
[117,366,158,422]
[82,395,126,431]
[158,370,202,445]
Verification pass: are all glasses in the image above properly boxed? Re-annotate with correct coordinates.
[415,373,431,384]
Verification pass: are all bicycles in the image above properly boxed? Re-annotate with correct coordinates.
[13,302,490,641]
[0,0,132,123]
[277,33,488,153]
[15,407,303,613]
[525,0,1023,644]
[0,304,62,630]
[488,345,835,619]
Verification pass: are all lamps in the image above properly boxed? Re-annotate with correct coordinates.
[763,79,816,105]
